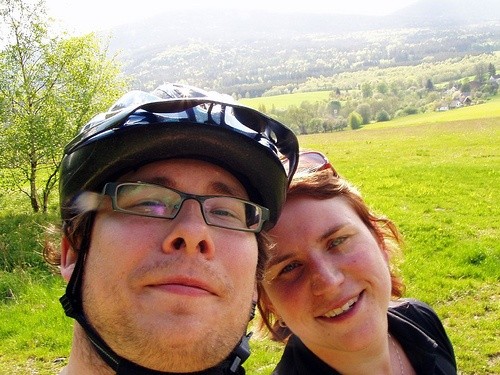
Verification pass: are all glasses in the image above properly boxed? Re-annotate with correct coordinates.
[280,151,341,179]
[102,181,271,233]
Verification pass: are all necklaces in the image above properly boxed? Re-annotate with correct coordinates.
[388,334,403,375]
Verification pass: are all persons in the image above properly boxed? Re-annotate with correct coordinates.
[38,80,300,375]
[256,148,458,375]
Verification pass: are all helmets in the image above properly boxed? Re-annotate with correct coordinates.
[59,84,299,224]
